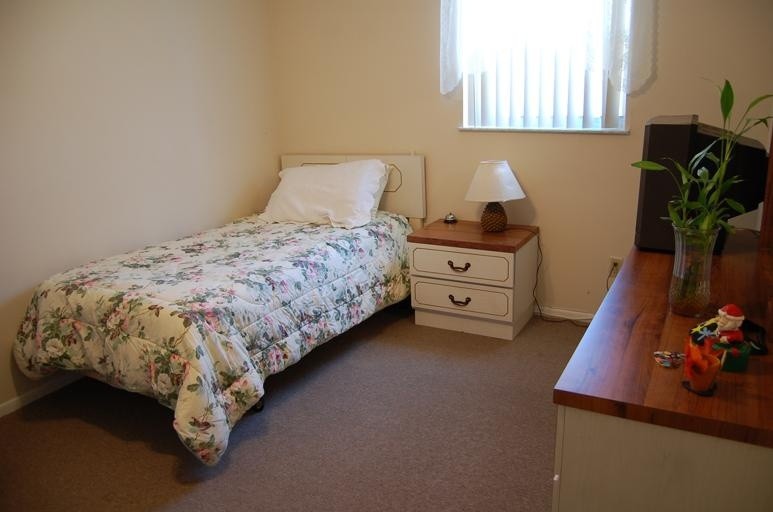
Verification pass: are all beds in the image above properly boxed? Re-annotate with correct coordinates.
[12,152,428,467]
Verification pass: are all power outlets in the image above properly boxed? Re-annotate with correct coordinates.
[609,256,622,277]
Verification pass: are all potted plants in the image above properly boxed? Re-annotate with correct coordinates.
[630,79,773,314]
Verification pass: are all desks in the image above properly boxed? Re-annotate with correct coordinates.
[553,222,772,512]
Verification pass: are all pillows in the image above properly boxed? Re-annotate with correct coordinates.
[263,160,395,228]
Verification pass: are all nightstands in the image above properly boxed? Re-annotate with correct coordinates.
[407,217,540,341]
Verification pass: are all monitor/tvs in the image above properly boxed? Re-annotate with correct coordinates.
[633,113,769,252]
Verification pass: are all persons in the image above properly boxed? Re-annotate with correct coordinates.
[688,302,750,372]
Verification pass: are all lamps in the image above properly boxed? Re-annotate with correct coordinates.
[465,158,526,230]
